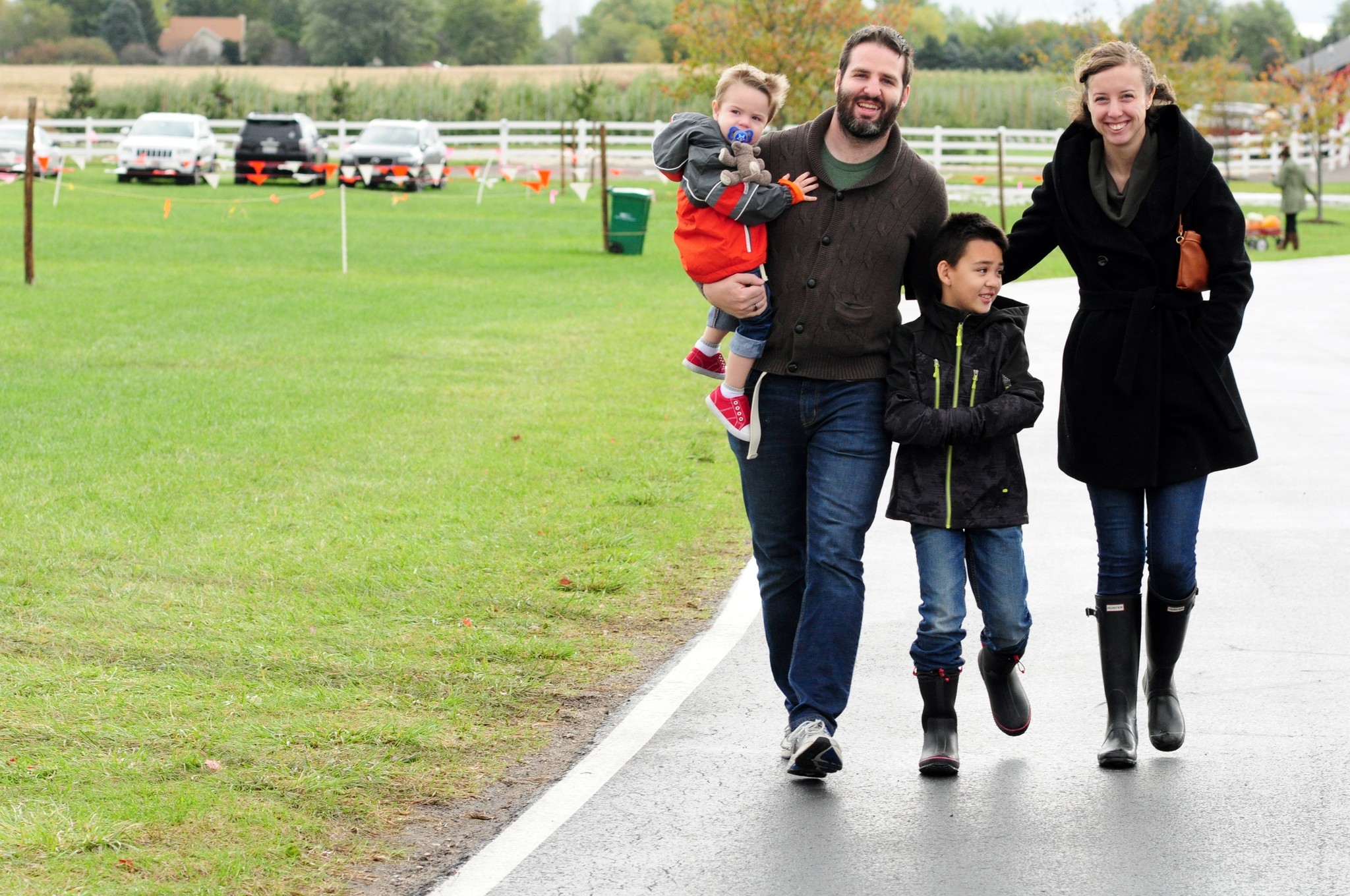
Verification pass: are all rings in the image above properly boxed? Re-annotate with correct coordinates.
[753,303,757,311]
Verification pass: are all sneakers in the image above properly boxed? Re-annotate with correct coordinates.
[780,725,792,760]
[682,348,726,380]
[705,385,750,441]
[786,718,843,779]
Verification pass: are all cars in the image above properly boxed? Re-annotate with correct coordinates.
[233,111,328,187]
[1,119,65,178]
[116,111,219,185]
[337,117,451,191]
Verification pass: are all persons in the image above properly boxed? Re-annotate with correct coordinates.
[883,212,1044,776]
[652,62,817,441]
[726,26,949,781]
[1003,42,1259,766]
[1271,146,1320,249]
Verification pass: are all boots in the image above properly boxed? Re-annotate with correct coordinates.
[1288,233,1299,250]
[1142,576,1199,752]
[978,635,1031,736]
[1086,594,1141,768]
[1276,235,1291,250]
[913,667,960,777]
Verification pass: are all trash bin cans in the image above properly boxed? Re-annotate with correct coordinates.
[605,187,655,255]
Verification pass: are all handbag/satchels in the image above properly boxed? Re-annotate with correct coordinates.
[1176,230,1209,292]
